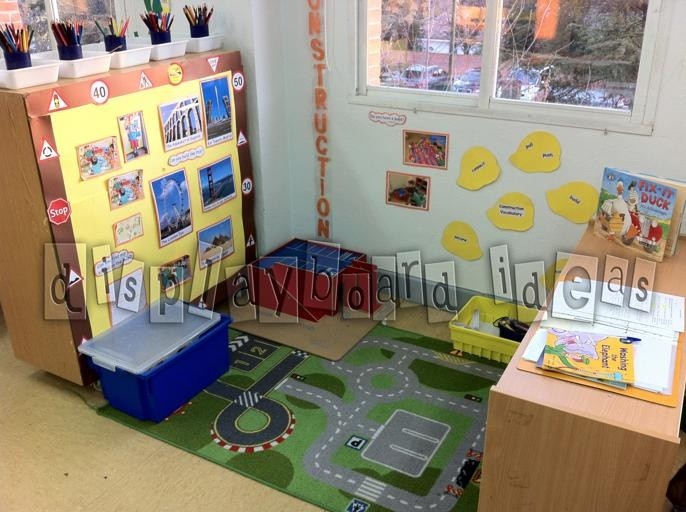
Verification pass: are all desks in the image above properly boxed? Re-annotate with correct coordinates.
[477,219,685,512]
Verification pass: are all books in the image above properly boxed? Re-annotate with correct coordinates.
[536,344,629,390]
[542,325,636,384]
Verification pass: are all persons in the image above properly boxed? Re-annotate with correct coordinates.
[76,108,150,207]
[159,253,194,292]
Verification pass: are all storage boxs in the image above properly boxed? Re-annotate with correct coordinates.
[76,295,233,424]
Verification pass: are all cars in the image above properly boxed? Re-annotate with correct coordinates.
[381,62,633,111]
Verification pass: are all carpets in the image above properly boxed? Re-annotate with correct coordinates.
[28,297,508,512]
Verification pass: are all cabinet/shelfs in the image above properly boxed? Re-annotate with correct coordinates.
[1,51,243,385]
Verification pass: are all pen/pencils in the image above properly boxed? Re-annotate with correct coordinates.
[95,17,130,39]
[182,1,214,25]
[0,21,37,55]
[49,19,83,45]
[138,8,175,33]
